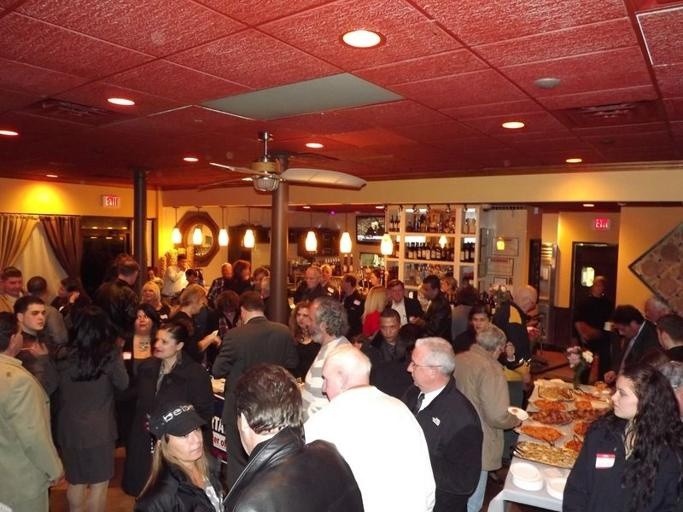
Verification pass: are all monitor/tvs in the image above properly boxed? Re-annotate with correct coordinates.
[356,215,384,243]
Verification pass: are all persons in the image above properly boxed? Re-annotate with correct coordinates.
[1,240,681,511]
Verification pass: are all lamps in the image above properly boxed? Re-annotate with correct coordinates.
[494,235,505,250]
[170,203,393,257]
[252,176,279,192]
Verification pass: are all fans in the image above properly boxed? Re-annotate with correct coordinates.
[198,132,365,190]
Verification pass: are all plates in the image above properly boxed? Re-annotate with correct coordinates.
[562,438,583,447]
[510,405,528,421]
[531,399,577,412]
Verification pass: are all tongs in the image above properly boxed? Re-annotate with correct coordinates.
[572,434,583,449]
[560,388,573,399]
[509,444,526,457]
[542,434,555,446]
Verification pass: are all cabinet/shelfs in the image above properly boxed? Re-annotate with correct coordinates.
[387,209,474,289]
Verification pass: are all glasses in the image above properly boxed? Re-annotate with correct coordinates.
[406,359,448,369]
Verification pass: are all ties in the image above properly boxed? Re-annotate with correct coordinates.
[412,393,427,420]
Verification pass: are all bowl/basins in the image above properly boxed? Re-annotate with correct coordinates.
[509,462,567,500]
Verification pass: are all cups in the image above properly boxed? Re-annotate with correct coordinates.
[594,381,607,398]
[603,322,611,331]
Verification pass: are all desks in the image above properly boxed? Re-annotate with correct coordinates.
[486,381,604,512]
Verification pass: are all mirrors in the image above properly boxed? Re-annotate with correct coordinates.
[174,211,222,268]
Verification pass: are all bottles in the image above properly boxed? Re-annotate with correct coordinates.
[414,263,453,287]
[324,253,354,275]
[393,241,474,261]
[388,210,476,234]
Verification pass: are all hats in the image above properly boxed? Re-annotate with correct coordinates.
[148,401,209,440]
